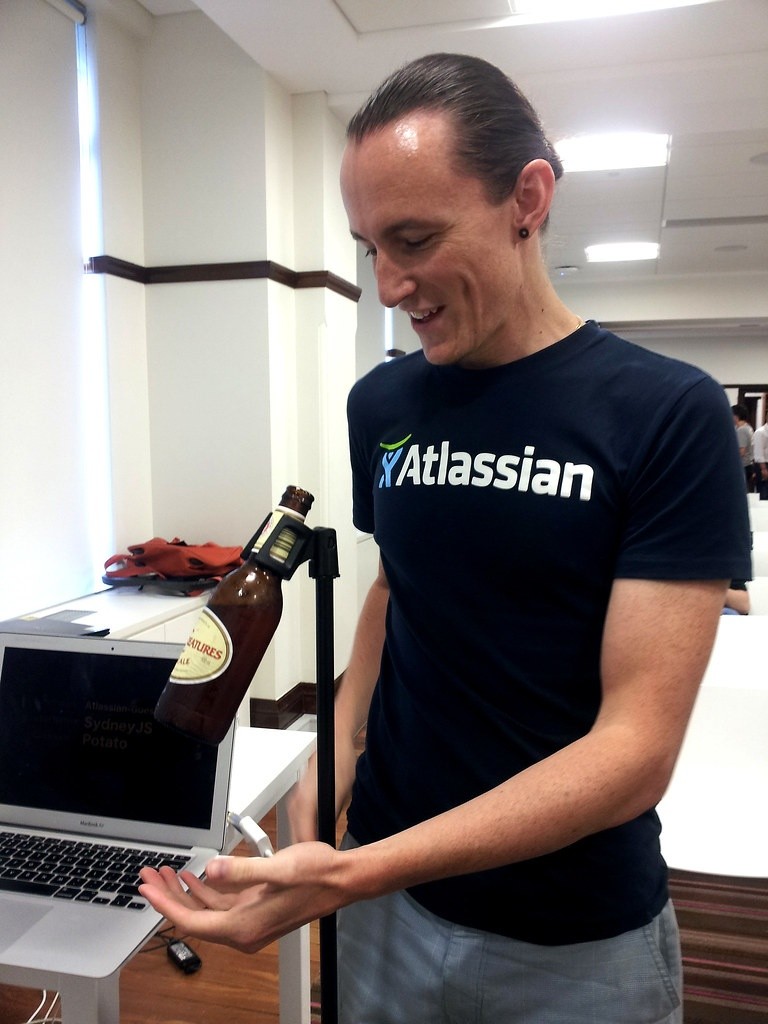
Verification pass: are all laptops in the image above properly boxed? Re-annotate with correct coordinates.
[0,633,238,978]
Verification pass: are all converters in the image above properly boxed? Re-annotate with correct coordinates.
[166,940,202,975]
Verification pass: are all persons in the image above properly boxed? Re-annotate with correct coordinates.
[136,52,752,1024]
[720,580,750,615]
[731,405,768,501]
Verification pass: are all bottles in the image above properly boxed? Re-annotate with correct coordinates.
[154,483,314,746]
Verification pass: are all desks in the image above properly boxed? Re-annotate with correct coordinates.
[1,724,320,1023]
